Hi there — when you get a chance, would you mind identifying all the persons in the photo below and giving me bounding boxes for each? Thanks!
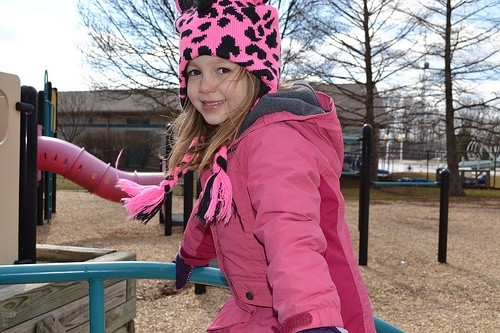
[117,1,379,333]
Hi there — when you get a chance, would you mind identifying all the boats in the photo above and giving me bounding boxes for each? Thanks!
[340,160,494,187]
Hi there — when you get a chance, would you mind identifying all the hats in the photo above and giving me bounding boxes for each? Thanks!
[115,2,281,226]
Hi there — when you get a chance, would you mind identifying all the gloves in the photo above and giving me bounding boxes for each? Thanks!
[172,254,210,295]
